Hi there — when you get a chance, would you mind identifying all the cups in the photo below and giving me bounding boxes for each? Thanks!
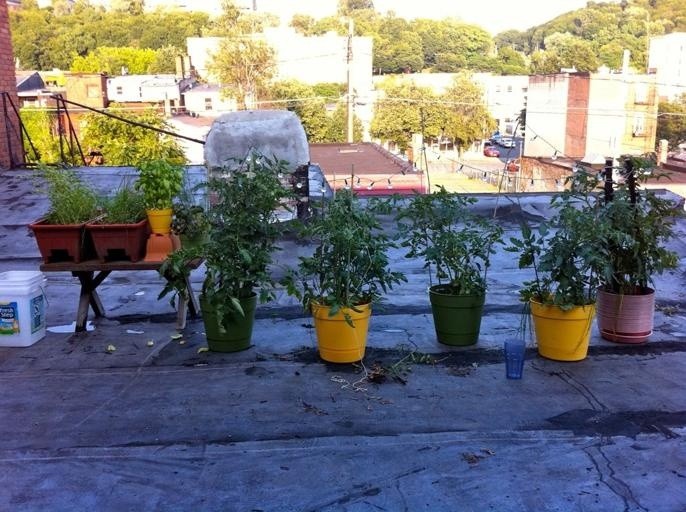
[502,336,527,380]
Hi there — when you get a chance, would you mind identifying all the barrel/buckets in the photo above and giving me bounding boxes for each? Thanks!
[429,285,484,346]
[596,286,655,344]
[530,296,596,361]
[0,271,49,348]
[310,299,372,364]
[199,291,258,352]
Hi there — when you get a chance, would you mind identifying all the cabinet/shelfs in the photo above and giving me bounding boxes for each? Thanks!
[170,201,212,249]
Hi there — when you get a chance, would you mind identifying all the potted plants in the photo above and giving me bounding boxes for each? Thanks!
[503,156,686,361]
[136,141,189,233]
[388,183,507,346]
[281,186,409,364]
[155,147,293,353]
[27,160,148,263]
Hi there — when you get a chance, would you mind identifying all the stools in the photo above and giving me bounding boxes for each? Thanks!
[39,256,203,329]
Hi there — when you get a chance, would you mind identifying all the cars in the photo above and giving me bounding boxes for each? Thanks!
[484,134,515,157]
